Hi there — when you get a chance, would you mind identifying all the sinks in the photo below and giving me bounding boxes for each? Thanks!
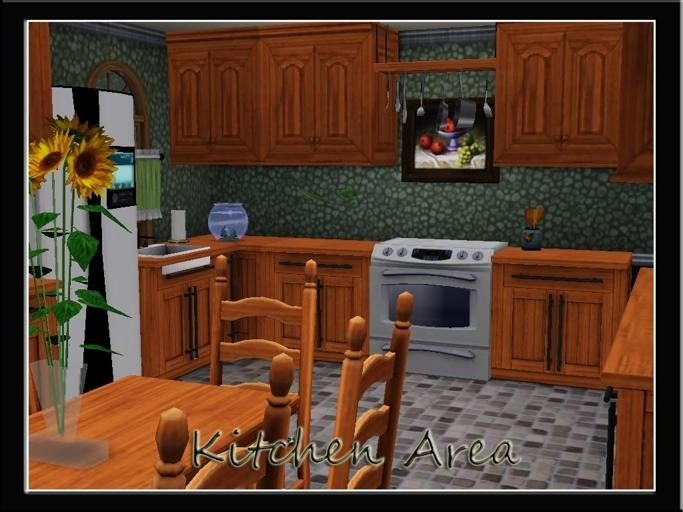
[137,243,210,258]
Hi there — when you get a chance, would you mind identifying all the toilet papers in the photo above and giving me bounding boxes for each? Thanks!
[171,210,187,241]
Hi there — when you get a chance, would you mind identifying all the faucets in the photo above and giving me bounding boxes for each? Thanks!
[137,223,140,249]
